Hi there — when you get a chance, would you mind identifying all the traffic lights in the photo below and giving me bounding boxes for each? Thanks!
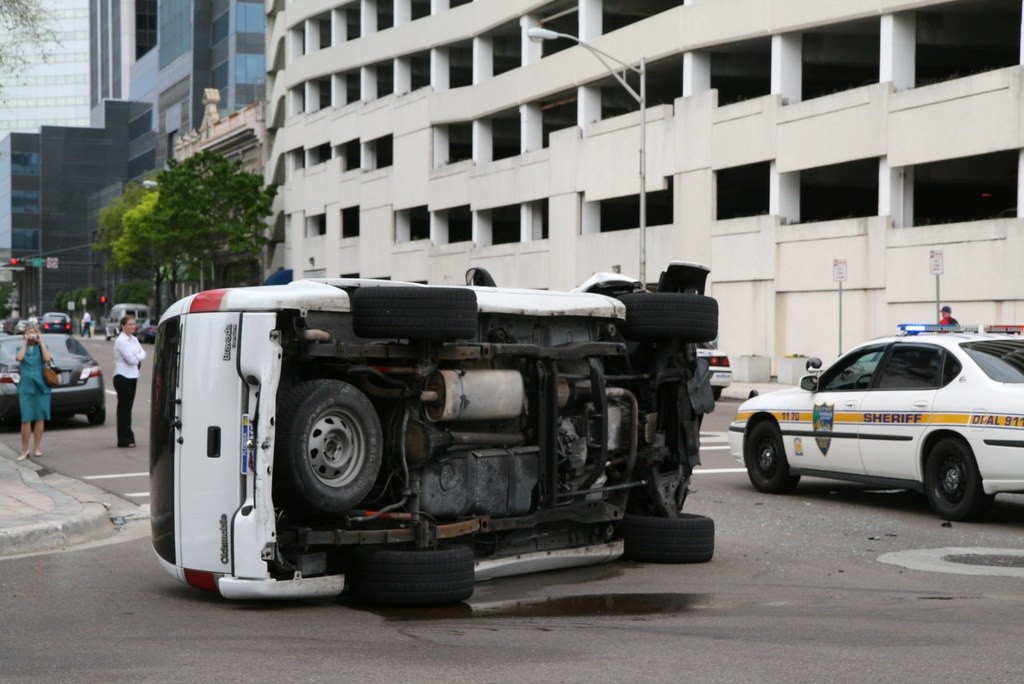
[10,258,26,266]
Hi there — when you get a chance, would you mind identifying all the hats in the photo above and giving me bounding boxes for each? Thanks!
[940,306,951,312]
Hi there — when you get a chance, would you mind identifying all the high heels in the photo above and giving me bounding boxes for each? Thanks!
[33,452,42,456]
[17,450,29,461]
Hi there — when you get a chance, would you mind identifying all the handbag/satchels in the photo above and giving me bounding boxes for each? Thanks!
[42,367,59,387]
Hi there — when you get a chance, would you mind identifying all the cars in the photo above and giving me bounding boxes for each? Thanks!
[0,334,106,432]
[729,323,1024,521]
[0,311,72,335]
[136,325,158,342]
[147,260,733,609]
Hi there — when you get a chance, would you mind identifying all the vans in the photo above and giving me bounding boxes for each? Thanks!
[105,303,151,339]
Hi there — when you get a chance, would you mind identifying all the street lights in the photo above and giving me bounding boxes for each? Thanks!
[527,28,646,295]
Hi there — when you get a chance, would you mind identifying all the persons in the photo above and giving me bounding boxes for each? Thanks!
[28,312,38,325]
[81,310,91,338]
[17,323,52,461]
[112,315,146,448]
[935,306,963,334]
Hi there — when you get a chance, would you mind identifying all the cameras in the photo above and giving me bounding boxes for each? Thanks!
[30,334,37,340]
